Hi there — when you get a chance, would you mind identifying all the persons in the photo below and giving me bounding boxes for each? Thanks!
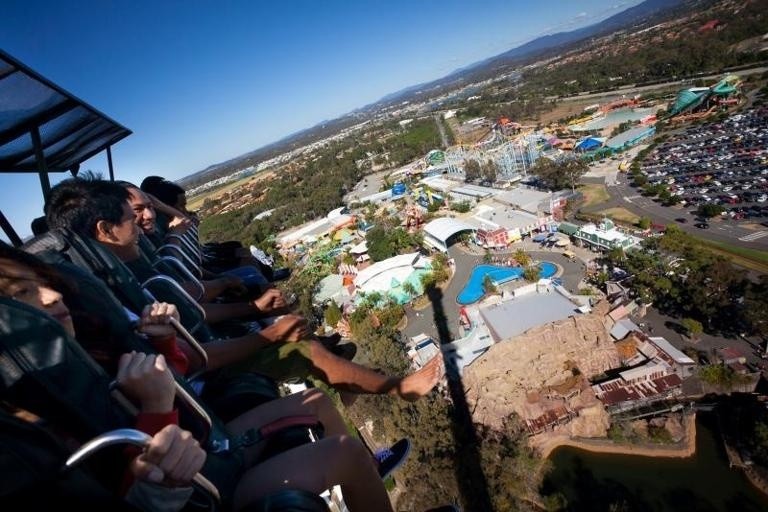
[1,173,442,511]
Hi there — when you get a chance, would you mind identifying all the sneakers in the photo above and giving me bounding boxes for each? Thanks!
[371,433,415,482]
[316,331,343,349]
[422,502,459,512]
[269,267,294,282]
[318,342,359,363]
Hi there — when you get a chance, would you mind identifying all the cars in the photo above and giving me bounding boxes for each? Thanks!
[533,231,571,250]
[585,151,631,167]
[611,268,627,282]
[638,83,768,230]
